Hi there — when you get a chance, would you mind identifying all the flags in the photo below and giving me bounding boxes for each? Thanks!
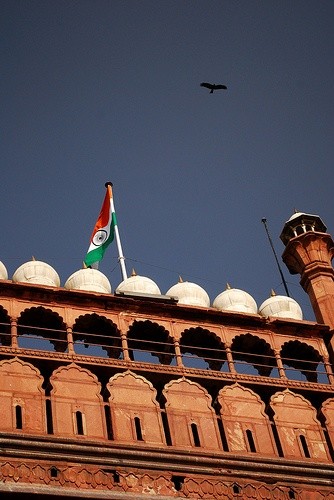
[84,184,118,270]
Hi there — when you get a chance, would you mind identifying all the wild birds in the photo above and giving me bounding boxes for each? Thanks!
[200,82,227,94]
